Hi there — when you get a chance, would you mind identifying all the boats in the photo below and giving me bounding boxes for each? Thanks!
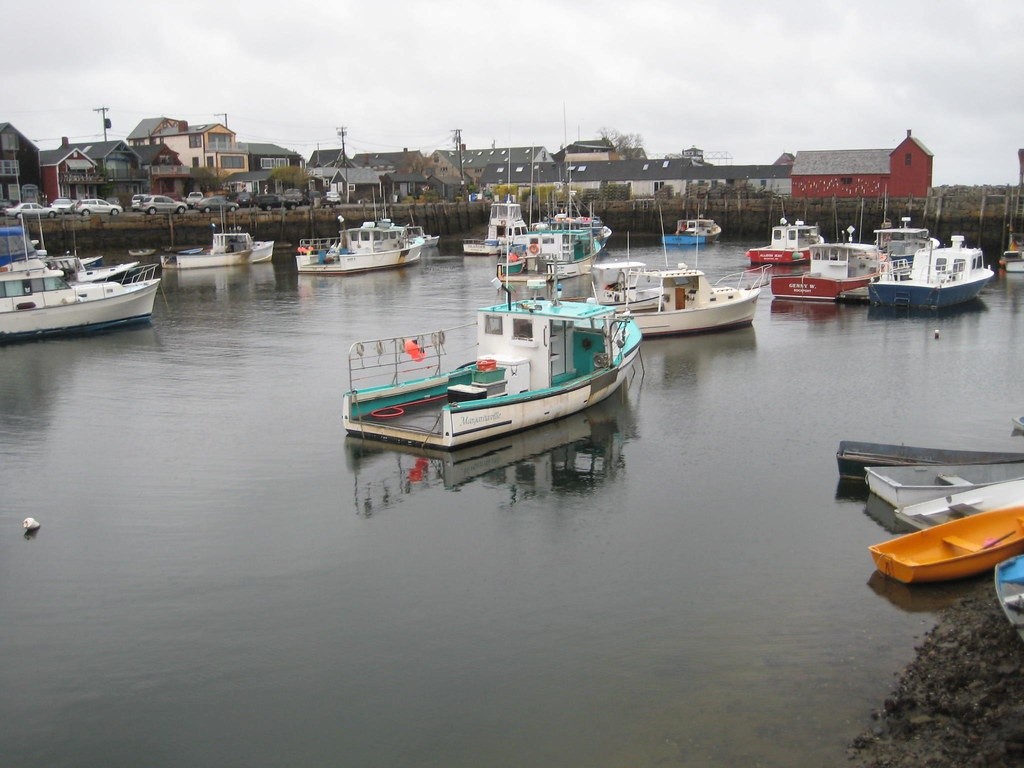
[0,257,141,288]
[836,440,1024,643]
[346,378,631,487]
[868,182,941,265]
[341,101,642,452]
[867,234,995,309]
[769,192,889,301]
[461,97,613,284]
[528,202,772,338]
[160,203,274,269]
[0,133,161,344]
[662,219,721,245]
[401,204,440,248]
[749,196,824,266]
[171,247,204,258]
[0,148,103,273]
[129,248,156,256]
[296,183,424,274]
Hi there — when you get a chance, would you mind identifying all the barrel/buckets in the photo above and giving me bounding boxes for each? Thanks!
[475,360,497,372]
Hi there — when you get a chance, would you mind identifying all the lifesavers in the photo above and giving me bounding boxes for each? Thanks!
[529,244,539,254]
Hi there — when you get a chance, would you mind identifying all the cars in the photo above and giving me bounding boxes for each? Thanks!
[0,190,342,218]
